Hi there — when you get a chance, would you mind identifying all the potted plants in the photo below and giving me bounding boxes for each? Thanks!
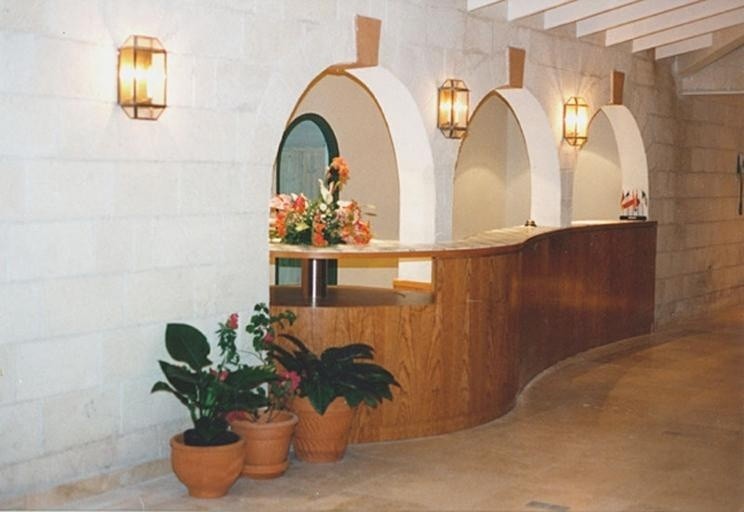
[139,317,248,497]
[272,332,403,466]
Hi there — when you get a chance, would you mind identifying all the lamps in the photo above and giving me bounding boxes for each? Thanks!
[562,95,587,145]
[114,33,170,122]
[436,78,471,141]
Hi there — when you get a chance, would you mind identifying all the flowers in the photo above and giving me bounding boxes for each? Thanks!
[264,154,373,248]
[210,297,304,425]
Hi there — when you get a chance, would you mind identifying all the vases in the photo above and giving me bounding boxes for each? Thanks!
[227,410,301,479]
